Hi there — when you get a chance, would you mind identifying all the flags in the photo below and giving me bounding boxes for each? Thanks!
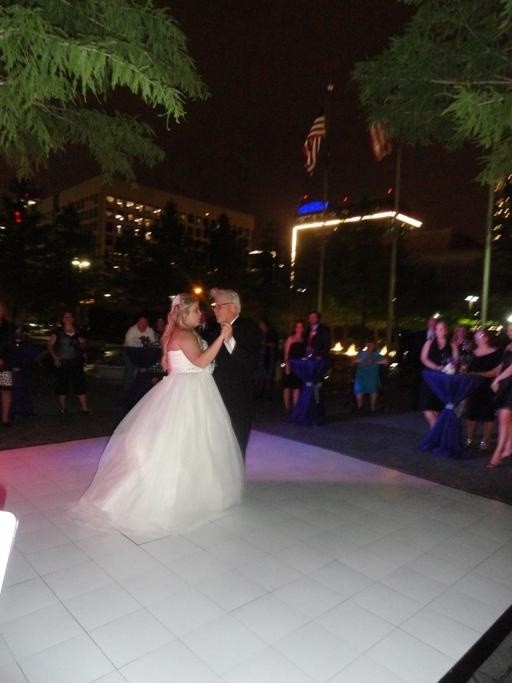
[368,117,393,160]
[303,85,326,177]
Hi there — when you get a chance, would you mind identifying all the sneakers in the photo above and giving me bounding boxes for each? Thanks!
[1,407,96,431]
[465,438,511,469]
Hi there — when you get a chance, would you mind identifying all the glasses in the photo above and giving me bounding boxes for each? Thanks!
[209,300,238,310]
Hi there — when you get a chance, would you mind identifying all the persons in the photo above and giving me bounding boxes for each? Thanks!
[1,300,20,424]
[68,291,246,535]
[417,315,511,470]
[47,310,91,415]
[122,312,389,425]
[165,289,262,468]
